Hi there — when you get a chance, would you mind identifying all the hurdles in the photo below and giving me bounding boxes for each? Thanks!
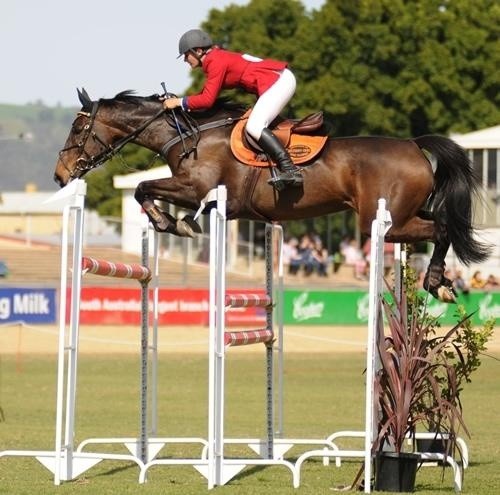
[1,178,468,493]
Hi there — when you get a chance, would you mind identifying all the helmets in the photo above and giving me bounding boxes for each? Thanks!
[177,29,212,59]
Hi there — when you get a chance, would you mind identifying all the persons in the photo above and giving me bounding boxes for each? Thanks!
[162,29,304,187]
[281,233,499,294]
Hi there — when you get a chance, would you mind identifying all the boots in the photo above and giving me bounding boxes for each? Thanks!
[256,128,304,187]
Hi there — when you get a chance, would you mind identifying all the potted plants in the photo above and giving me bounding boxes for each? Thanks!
[327,243,500,493]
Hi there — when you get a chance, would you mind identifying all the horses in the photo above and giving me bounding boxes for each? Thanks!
[53,87,497,304]
